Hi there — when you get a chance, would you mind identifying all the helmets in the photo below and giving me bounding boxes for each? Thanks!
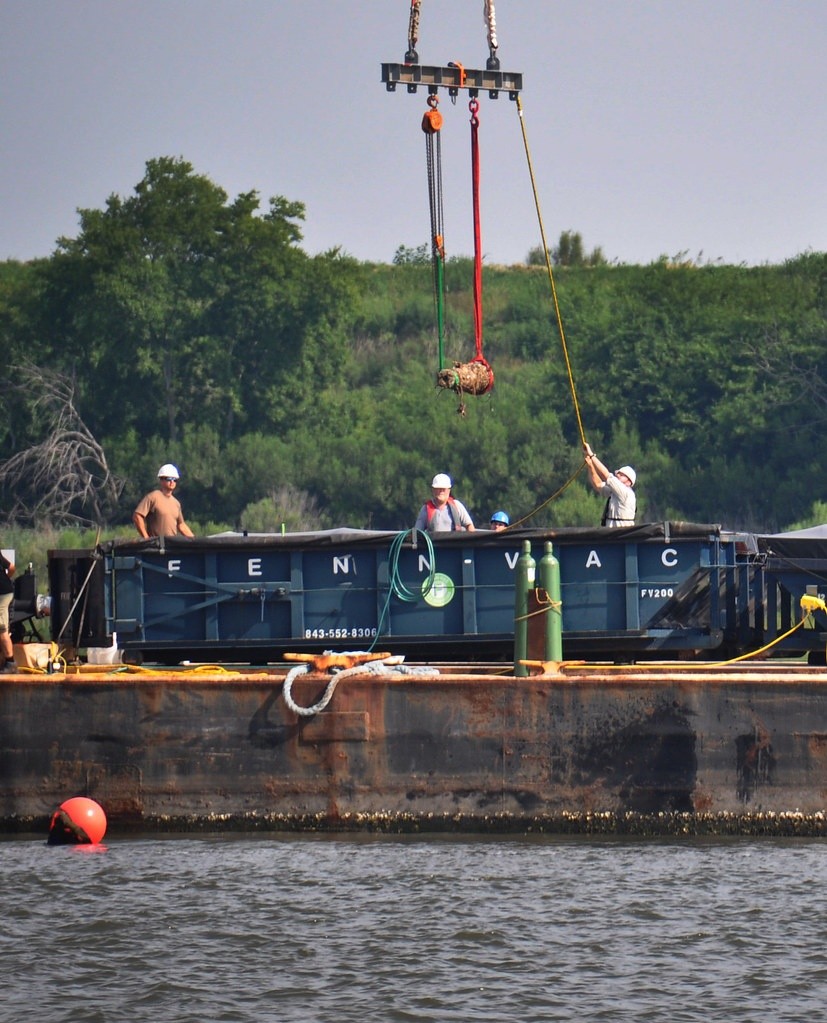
[615,465,637,487]
[490,511,509,525]
[432,473,452,489]
[157,464,180,479]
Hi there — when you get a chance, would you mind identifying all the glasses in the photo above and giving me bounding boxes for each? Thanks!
[162,477,177,482]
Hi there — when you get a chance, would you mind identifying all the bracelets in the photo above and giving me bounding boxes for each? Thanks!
[590,454,596,459]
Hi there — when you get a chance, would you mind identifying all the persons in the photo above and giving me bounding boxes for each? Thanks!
[0,551,19,674]
[491,512,509,530]
[582,443,636,527]
[414,474,475,531]
[133,464,195,537]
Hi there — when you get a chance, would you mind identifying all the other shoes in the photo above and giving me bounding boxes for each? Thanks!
[0,661,18,673]
